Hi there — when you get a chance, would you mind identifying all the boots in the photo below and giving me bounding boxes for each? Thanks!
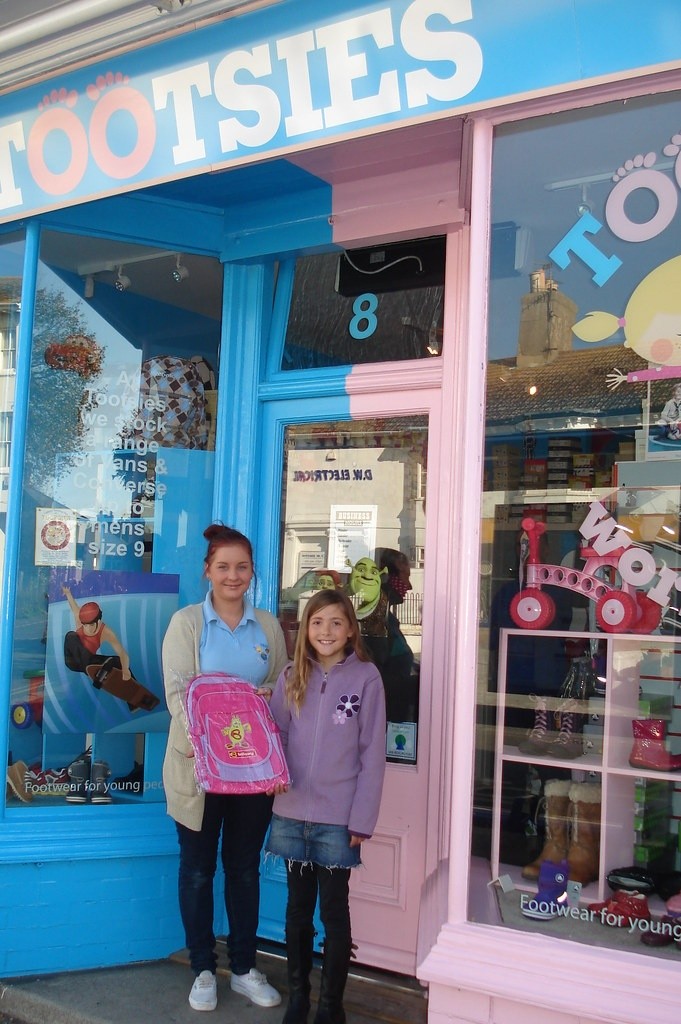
[282,930,318,1024]
[313,937,357,1024]
[499,657,681,951]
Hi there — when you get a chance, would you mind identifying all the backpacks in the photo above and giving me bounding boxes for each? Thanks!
[186,672,290,793]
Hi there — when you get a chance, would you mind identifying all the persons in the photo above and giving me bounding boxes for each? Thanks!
[358,547,417,725]
[162,521,286,1011]
[660,384,681,440]
[266,589,387,1024]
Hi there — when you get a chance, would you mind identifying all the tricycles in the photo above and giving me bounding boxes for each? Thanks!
[510,517,662,635]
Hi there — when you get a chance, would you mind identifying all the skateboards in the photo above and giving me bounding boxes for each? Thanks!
[85,656,161,711]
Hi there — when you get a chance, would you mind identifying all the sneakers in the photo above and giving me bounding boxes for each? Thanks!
[188,970,217,1010]
[231,968,281,1007]
[7,759,144,803]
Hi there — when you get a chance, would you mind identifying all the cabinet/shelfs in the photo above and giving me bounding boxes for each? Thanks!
[491,628,681,918]
[42,446,216,803]
